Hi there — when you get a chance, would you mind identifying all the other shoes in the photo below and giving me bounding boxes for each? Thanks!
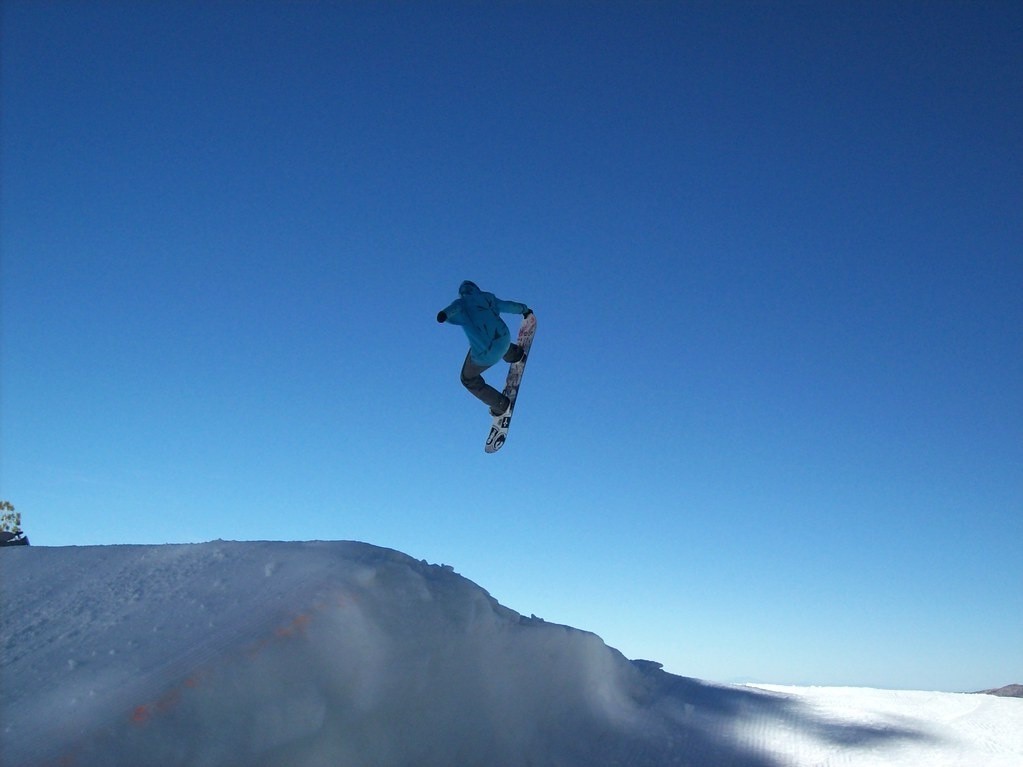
[488,407,503,417]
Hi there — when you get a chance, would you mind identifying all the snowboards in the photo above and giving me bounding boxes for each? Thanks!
[484,312,537,454]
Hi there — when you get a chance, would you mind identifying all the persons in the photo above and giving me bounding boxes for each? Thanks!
[436,279,533,420]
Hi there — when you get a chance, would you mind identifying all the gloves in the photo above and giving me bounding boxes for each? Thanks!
[437,311,448,323]
[523,308,534,319]
